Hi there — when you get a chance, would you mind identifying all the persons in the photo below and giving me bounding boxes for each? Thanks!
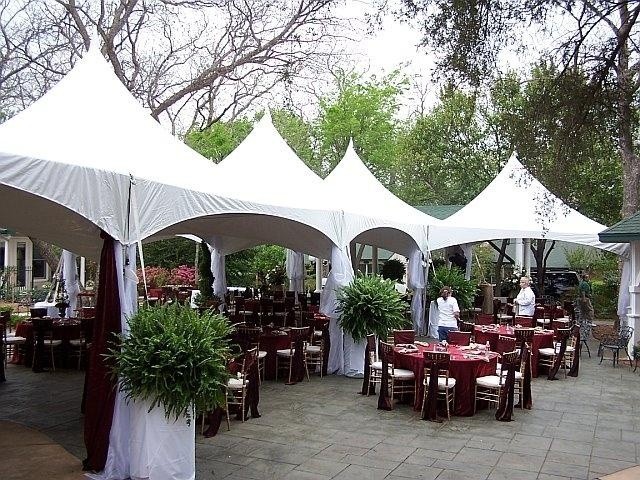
[512,276,536,317]
[575,273,598,328]
[436,285,461,341]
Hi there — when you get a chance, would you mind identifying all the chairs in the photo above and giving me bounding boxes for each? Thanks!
[0,285,196,372]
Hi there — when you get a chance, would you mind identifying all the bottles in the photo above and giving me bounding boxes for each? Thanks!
[485,340,491,352]
